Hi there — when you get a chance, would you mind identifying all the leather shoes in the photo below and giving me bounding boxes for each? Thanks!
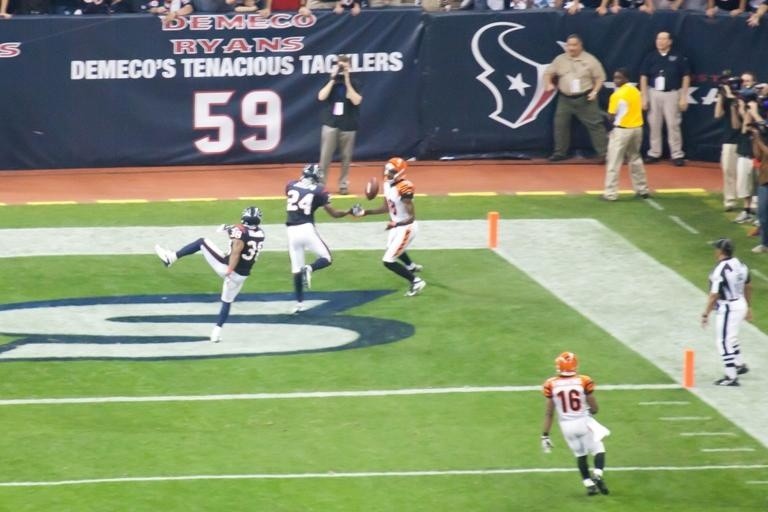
[676,159,684,166]
[595,155,606,163]
[643,155,660,163]
[548,153,569,161]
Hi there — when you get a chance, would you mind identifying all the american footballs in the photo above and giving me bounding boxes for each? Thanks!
[365,177,379,200]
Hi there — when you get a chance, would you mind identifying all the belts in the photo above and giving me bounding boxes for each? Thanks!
[654,87,678,93]
[558,88,594,100]
[611,125,625,129]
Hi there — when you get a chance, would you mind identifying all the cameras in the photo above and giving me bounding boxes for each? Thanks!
[338,65,345,73]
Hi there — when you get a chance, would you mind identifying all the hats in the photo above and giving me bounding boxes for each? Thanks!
[711,237,733,258]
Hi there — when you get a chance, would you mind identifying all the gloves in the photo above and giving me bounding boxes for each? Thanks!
[226,272,234,287]
[349,202,367,217]
[383,220,396,233]
[541,436,555,454]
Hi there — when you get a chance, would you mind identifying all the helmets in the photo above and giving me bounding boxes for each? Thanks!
[304,164,321,183]
[382,156,408,185]
[555,351,578,377]
[240,207,262,226]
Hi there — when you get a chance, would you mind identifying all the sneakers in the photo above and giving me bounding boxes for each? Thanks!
[753,219,760,227]
[732,210,753,224]
[405,275,426,297]
[748,225,762,236]
[592,473,610,495]
[715,376,740,387]
[751,244,768,254]
[292,303,306,314]
[724,362,748,376]
[583,478,600,496]
[404,263,423,274]
[153,243,176,268]
[211,326,222,343]
[300,265,312,289]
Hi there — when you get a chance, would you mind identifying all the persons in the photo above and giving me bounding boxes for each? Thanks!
[602,67,650,200]
[700,239,752,385]
[637,30,692,165]
[733,83,768,223]
[156,207,265,343]
[4,1,767,28]
[355,157,425,297]
[285,164,361,316]
[316,54,364,195]
[541,352,612,496]
[712,73,759,211]
[749,125,768,252]
[542,32,609,164]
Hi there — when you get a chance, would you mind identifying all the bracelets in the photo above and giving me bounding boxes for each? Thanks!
[329,75,337,80]
[701,314,708,318]
[543,432,549,436]
[396,222,400,226]
[590,90,600,97]
